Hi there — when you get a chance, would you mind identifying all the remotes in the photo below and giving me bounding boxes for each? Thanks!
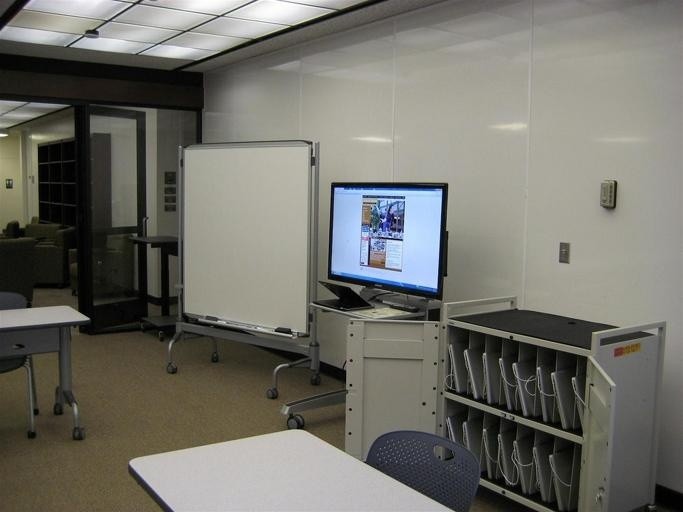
[390,302,419,313]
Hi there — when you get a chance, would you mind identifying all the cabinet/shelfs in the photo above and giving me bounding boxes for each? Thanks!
[344,293,669,512]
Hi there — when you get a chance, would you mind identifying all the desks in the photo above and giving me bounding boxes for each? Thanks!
[1,304,95,442]
[129,428,455,512]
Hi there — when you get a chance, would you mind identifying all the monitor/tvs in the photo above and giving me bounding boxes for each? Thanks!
[328,181,448,301]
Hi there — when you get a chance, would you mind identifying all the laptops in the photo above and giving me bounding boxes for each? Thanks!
[313,280,375,311]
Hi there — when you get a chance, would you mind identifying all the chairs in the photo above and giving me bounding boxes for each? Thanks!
[361,430,482,512]
[0,292,40,439]
[0,222,135,298]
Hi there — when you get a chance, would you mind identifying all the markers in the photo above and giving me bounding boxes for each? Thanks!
[205,316,218,322]
[275,326,294,335]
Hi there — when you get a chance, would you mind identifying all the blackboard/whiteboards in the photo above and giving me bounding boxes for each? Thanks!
[179,140,312,338]
